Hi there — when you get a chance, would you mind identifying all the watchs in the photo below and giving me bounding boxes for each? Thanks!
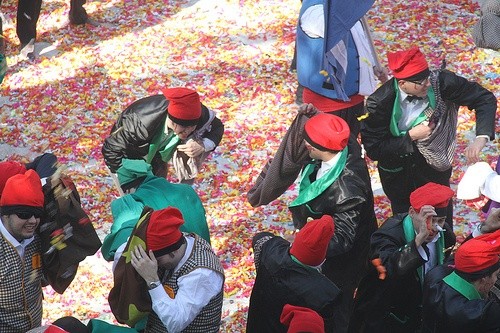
[150,281,161,290]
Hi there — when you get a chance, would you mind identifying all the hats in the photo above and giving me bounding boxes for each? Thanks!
[386,46,430,81]
[303,113,349,151]
[290,215,335,266]
[0,160,28,195]
[456,162,496,211]
[163,87,201,126]
[280,305,324,333]
[455,228,500,279]
[146,206,185,258]
[410,182,454,217]
[0,169,45,208]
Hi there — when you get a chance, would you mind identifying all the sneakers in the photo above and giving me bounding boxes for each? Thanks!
[20,42,34,59]
[84,18,101,28]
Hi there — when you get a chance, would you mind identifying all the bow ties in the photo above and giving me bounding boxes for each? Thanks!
[407,94,423,104]
[422,242,430,260]
[308,161,321,183]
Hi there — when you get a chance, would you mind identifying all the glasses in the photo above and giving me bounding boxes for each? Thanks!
[406,76,428,90]
[7,207,42,219]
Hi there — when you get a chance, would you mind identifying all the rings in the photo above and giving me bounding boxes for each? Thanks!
[138,257,141,260]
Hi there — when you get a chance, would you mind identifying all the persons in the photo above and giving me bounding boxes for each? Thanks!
[0,160,103,333]
[130,206,225,333]
[370,182,456,333]
[246,186,500,333]
[360,47,497,229]
[16,0,98,61]
[101,89,224,195]
[288,103,378,254]
[297,0,386,137]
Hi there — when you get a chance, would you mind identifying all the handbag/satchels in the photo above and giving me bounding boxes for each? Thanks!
[172,110,216,186]
[414,70,458,172]
